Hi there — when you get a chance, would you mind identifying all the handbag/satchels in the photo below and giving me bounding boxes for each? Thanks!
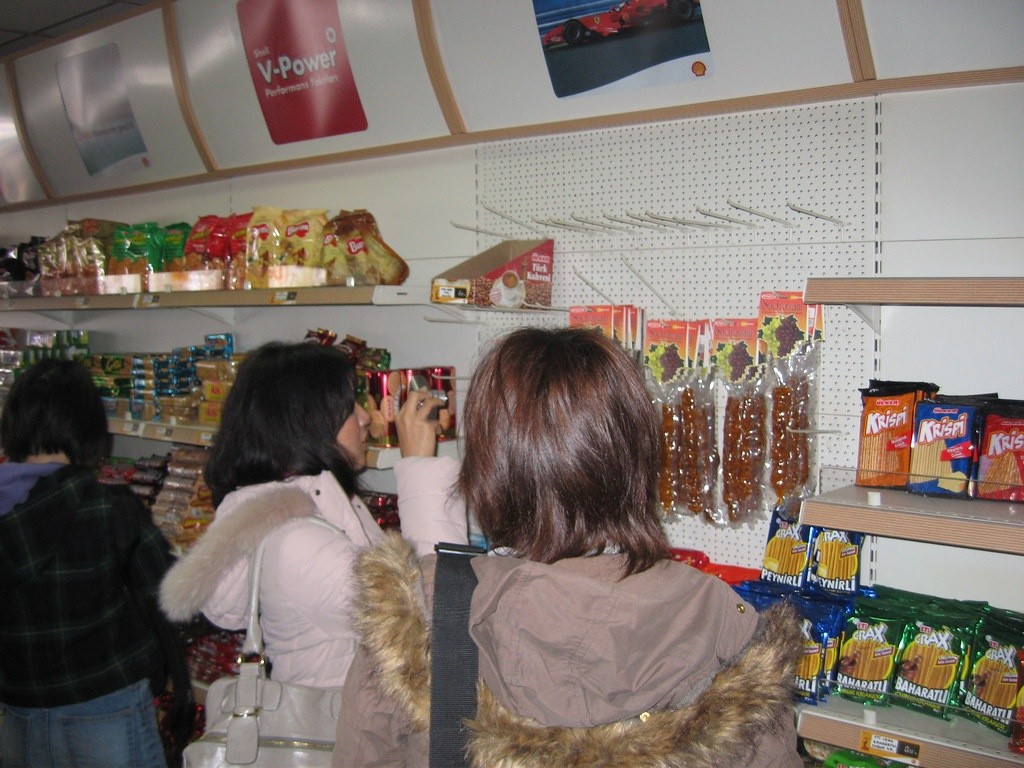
[182,515,362,768]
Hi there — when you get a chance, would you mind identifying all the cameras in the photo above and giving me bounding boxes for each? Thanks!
[408,374,449,423]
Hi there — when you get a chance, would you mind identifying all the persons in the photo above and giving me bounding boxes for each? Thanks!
[157,336,471,688]
[329,323,808,767]
[1,356,184,768]
